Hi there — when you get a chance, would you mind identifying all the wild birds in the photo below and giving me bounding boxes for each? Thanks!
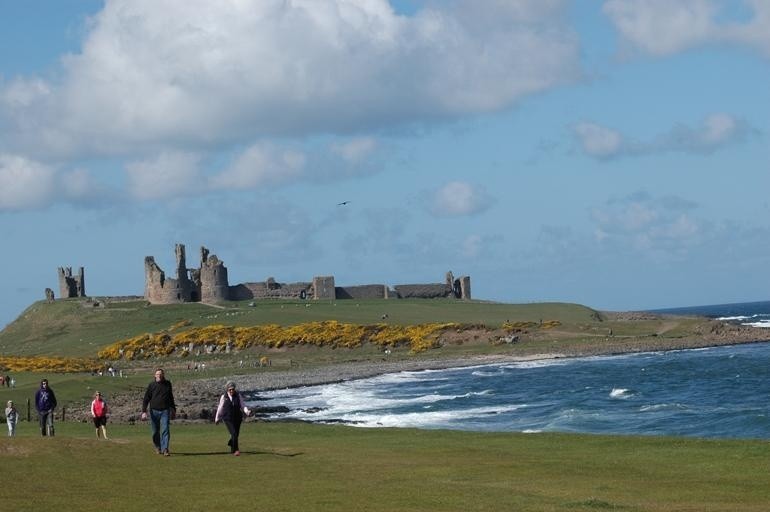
[338,201,351,205]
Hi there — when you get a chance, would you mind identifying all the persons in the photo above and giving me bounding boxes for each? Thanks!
[239,359,244,369]
[140,368,177,456]
[90,363,128,378]
[185,360,208,374]
[0,375,17,388]
[214,381,251,456]
[35,378,57,437]
[4,399,19,438]
[91,390,110,440]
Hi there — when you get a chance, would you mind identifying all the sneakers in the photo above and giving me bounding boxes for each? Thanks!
[156,448,161,454]
[164,451,170,456]
[233,451,240,456]
